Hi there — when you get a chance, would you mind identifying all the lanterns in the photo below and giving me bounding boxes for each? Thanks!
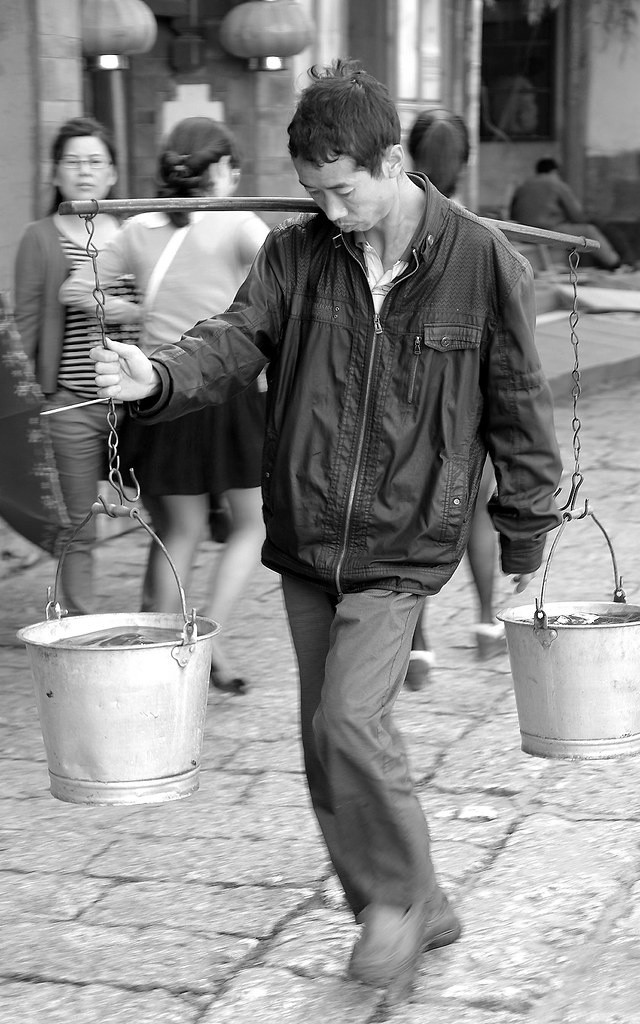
[81,0,157,71]
[219,0,313,72]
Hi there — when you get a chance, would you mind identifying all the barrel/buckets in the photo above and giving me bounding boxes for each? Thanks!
[494,504,640,761]
[15,503,222,806]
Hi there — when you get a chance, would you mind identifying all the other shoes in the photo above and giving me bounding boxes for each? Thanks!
[472,621,509,660]
[422,895,463,952]
[347,886,450,983]
[211,663,248,696]
[406,650,434,689]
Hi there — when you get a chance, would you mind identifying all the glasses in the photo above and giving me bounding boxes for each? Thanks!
[211,159,242,187]
[59,152,113,172]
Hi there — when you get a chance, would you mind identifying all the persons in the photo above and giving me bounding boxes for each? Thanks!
[508,160,627,274]
[90,58,565,992]
[57,116,271,695]
[14,118,145,619]
[406,108,508,691]
